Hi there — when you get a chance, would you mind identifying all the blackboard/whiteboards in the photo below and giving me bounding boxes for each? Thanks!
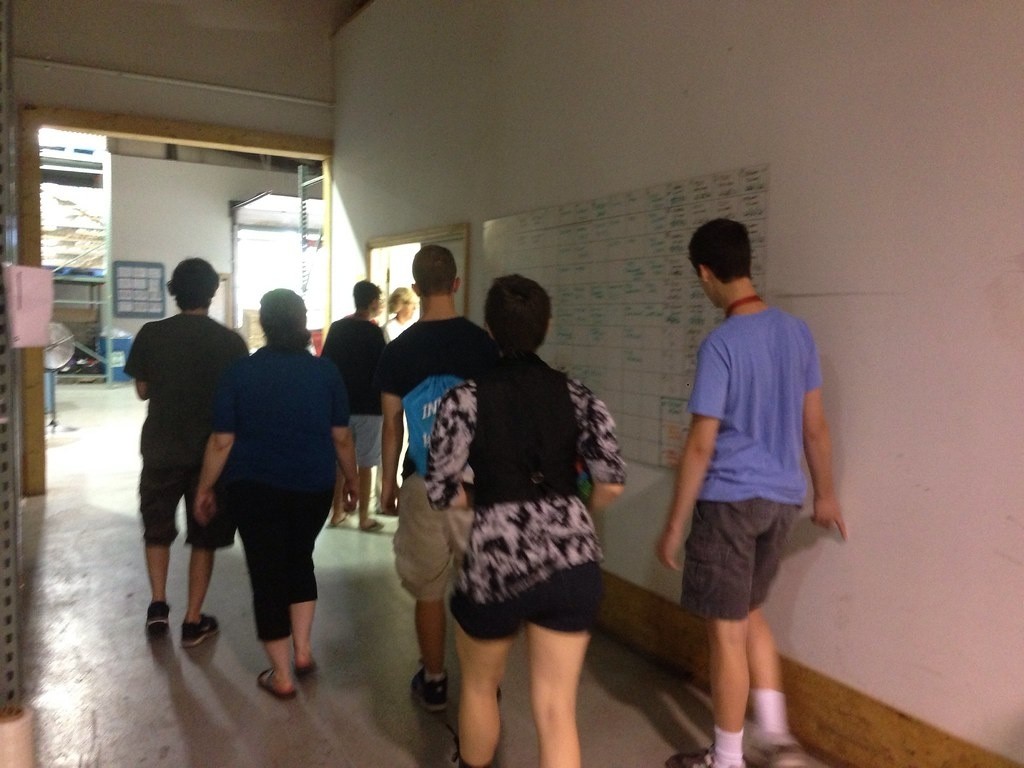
[482,163,768,474]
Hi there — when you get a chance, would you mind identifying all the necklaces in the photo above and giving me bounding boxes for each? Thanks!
[724,295,763,318]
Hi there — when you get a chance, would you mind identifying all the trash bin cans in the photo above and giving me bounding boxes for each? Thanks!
[99,337,133,382]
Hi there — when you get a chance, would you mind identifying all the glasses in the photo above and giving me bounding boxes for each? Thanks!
[375,298,384,304]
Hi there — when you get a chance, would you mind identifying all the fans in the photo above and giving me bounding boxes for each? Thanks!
[44,322,77,434]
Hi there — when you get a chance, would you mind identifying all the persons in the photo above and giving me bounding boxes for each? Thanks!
[192,288,363,699]
[377,245,505,708]
[655,218,848,768]
[425,273,626,768]
[317,279,416,533]
[121,259,249,649]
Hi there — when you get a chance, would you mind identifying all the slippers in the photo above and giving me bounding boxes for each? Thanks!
[361,519,385,531]
[327,513,352,527]
[294,657,316,677]
[258,668,297,698]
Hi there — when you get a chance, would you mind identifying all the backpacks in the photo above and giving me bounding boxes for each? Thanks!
[401,373,469,477]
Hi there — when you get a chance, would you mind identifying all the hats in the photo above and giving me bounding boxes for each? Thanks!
[258,287,307,325]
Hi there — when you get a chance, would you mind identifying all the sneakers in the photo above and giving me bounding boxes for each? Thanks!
[411,666,449,710]
[742,732,807,767]
[145,602,170,636]
[182,613,219,646]
[665,742,746,768]
